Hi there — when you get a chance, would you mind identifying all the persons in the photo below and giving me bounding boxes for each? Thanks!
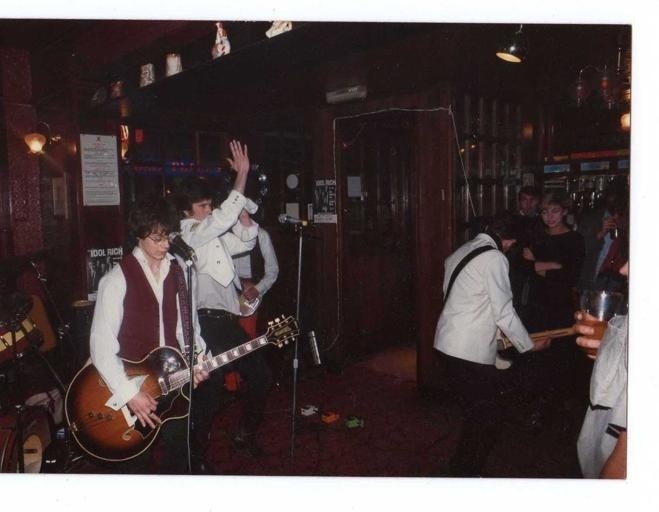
[221,176,282,396]
[170,138,276,475]
[429,176,627,479]
[87,192,211,474]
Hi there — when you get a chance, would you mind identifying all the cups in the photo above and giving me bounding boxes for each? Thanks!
[577,289,625,360]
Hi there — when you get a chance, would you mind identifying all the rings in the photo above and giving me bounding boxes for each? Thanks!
[148,411,154,416]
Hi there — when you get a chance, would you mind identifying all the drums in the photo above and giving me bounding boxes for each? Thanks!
[1,352,67,430]
[0,400,60,473]
[0,297,44,368]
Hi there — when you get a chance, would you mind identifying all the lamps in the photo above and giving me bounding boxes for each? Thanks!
[568,33,631,134]
[496,24,530,64]
[24,122,62,157]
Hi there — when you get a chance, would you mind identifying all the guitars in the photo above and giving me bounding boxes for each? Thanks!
[64,311,304,461]
[495,309,594,370]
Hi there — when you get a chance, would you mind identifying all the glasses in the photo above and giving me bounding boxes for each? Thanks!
[147,234,167,245]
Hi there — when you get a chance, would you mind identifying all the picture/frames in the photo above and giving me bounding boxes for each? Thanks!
[51,169,69,221]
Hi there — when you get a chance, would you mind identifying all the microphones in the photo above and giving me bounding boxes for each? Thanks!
[168,232,199,262]
[278,214,308,227]
